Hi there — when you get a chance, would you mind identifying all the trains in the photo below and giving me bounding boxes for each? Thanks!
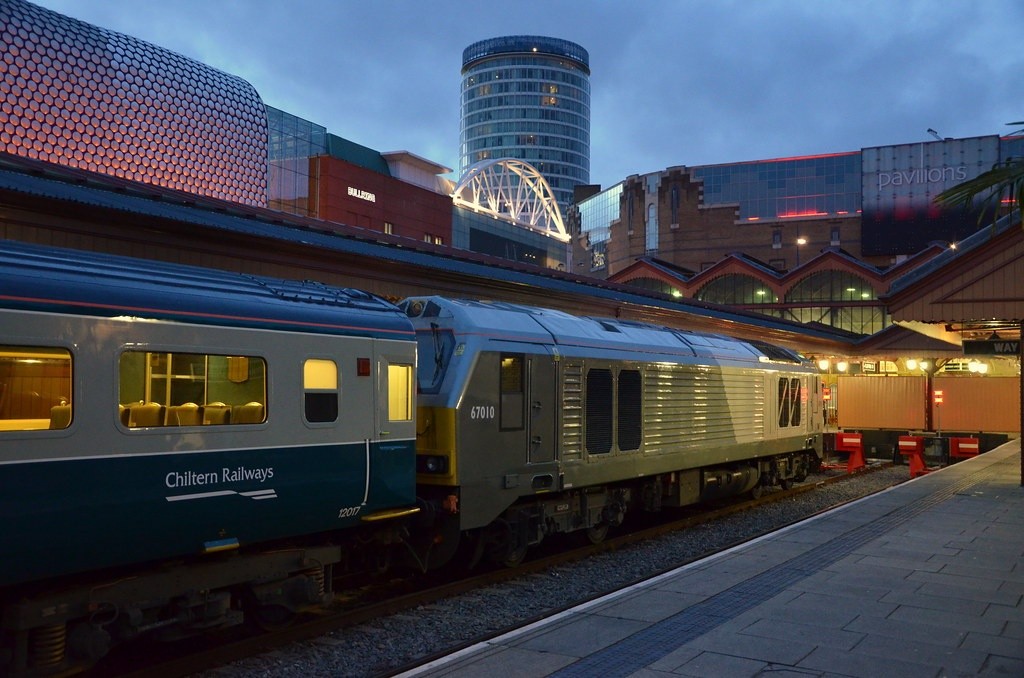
[1,241,826,678]
